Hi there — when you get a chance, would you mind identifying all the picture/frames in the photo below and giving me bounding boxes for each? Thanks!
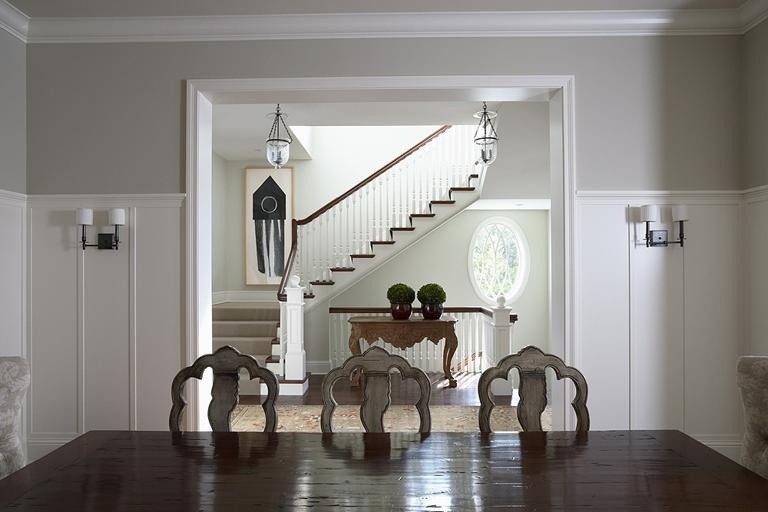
[243,166,295,285]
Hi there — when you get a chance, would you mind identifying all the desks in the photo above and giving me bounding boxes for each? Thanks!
[0,430,766,512]
[348,316,458,388]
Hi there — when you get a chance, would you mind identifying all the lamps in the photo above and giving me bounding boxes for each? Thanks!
[265,104,293,167]
[77,208,125,251]
[473,101,499,165]
[639,204,689,247]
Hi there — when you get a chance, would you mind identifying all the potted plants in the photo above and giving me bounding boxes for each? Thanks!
[387,283,414,319]
[417,283,446,319]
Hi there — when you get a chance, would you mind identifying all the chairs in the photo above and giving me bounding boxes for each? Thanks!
[477,346,590,433]
[319,346,431,433]
[0,355,32,478]
[735,356,767,479]
[168,345,278,434]
